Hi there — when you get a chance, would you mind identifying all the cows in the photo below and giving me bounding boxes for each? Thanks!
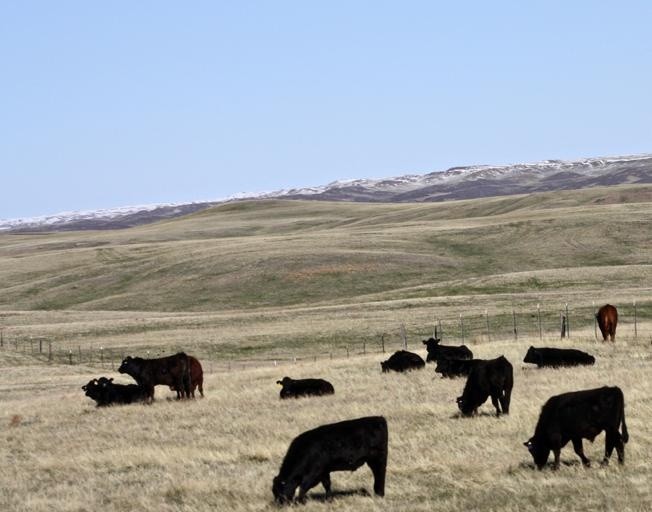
[522,385,629,471]
[270,414,389,505]
[80,352,206,409]
[380,349,426,373]
[456,355,515,417]
[275,376,335,399]
[595,303,618,341]
[435,358,489,379]
[422,337,474,363]
[522,345,596,368]
